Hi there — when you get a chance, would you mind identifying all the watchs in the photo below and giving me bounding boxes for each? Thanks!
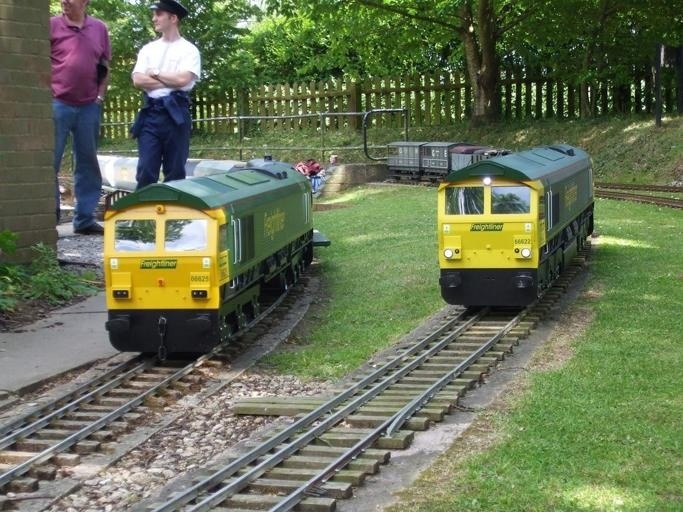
[97,95,105,102]
[153,69,160,78]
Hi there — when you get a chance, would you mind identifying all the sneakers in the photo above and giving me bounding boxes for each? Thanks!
[75,222,104,235]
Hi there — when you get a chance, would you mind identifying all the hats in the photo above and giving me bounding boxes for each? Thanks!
[147,0,189,20]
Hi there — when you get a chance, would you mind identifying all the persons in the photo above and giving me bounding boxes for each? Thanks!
[48,0,107,240]
[126,0,202,192]
